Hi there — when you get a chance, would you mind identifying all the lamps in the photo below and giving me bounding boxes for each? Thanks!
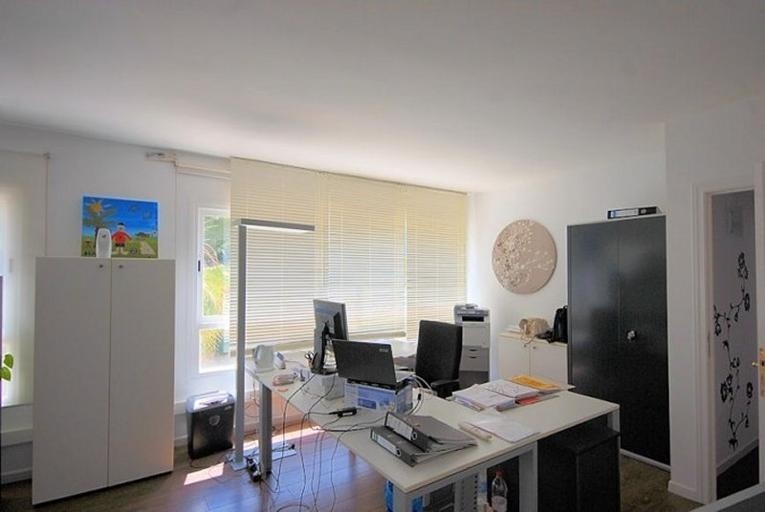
[226,214,319,473]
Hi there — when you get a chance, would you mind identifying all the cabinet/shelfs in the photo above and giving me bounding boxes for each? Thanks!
[493,329,569,382]
[29,247,181,509]
[479,417,622,511]
[454,319,492,390]
[563,211,676,475]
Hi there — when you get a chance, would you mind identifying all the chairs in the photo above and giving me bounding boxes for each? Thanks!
[410,316,466,400]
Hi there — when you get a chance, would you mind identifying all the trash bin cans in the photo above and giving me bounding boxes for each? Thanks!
[370,411,478,467]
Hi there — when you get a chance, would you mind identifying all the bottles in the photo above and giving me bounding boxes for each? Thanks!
[487,469,509,510]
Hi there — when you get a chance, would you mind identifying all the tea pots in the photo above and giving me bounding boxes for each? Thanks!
[251,345,276,371]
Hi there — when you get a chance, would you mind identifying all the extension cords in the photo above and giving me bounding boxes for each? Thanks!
[248,458,259,482]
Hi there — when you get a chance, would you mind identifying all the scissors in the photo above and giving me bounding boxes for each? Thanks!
[304,351,315,361]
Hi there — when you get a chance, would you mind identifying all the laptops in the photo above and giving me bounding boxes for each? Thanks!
[332,339,416,385]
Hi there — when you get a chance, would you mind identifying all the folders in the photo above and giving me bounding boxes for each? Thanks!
[607,206,662,218]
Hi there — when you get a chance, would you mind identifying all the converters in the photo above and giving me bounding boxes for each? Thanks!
[337,407,357,417]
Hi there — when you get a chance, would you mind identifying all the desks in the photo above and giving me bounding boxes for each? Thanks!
[246,345,628,512]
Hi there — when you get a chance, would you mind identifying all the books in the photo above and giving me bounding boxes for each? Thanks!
[452,375,576,412]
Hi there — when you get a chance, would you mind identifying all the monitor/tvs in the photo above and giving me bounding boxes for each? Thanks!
[311,299,348,375]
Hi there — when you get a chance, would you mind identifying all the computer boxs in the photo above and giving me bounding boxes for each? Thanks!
[186,389,235,460]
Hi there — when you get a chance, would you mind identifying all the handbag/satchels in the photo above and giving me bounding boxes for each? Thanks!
[553,305,568,343]
[520,318,550,335]
